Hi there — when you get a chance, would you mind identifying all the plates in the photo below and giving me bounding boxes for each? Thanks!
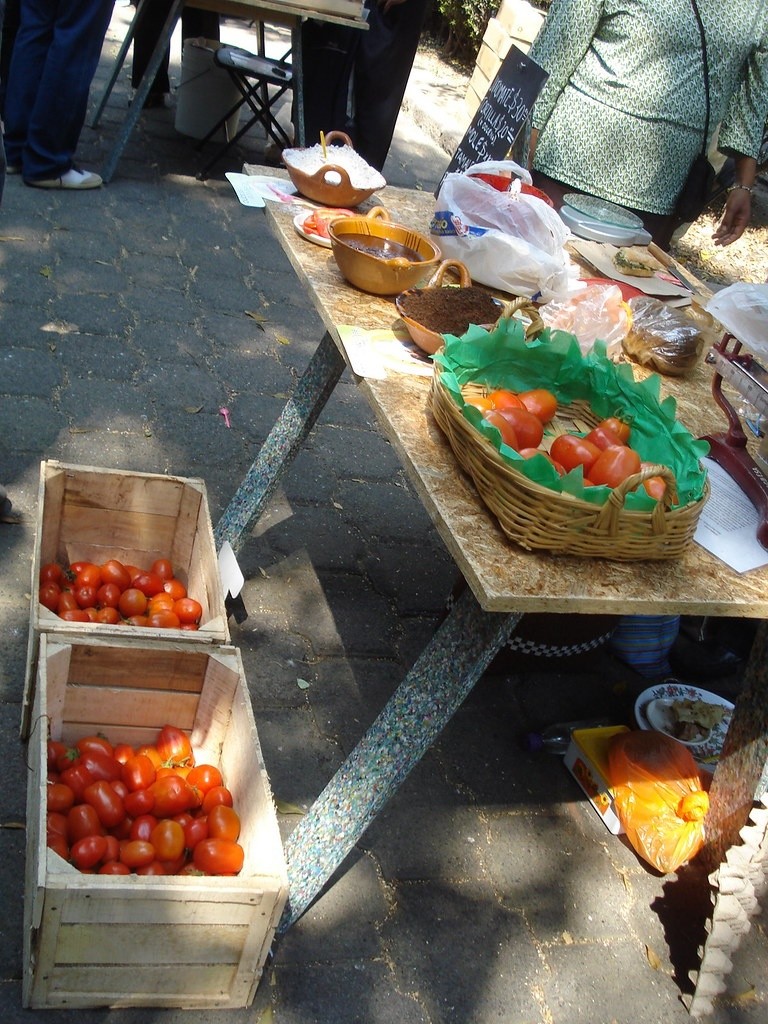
[635,684,735,763]
[648,699,712,745]
[293,213,358,248]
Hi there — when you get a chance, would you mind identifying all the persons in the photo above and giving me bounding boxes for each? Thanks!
[512,0,768,253]
[128,0,220,109]
[0,0,116,189]
[264,0,434,172]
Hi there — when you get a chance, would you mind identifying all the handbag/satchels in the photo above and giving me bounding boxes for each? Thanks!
[677,159,716,223]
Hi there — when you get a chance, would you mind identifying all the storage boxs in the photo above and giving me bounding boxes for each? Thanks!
[16,457,231,745]
[18,631,289,1008]
[566,725,634,836]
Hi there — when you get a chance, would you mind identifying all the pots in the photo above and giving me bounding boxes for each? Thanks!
[397,259,525,357]
[283,131,387,207]
[327,206,443,296]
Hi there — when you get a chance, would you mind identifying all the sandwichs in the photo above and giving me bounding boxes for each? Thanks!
[613,247,659,277]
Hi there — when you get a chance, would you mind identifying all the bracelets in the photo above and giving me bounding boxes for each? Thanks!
[727,184,753,195]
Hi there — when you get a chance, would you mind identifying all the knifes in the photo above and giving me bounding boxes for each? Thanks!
[648,244,702,295]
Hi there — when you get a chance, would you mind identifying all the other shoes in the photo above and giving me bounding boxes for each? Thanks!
[135,93,170,108]
[5,162,104,191]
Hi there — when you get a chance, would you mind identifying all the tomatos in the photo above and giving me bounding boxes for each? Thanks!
[304,206,358,240]
[462,389,682,507]
[44,724,246,877]
[39,558,203,632]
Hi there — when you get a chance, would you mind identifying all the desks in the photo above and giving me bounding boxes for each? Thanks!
[92,0,369,187]
[215,161,767,937]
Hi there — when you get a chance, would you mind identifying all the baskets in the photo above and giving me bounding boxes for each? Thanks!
[426,296,712,562]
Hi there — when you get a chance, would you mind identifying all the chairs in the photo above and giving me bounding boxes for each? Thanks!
[196,46,293,182]
